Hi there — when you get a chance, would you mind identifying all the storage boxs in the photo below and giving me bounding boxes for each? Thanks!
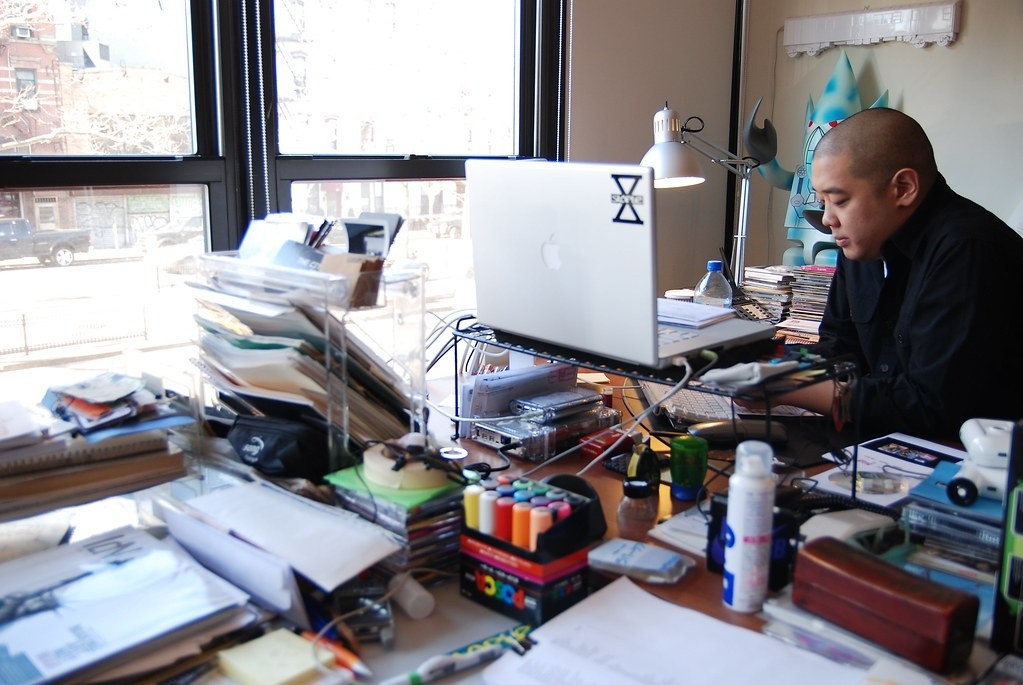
[580,424,650,462]
[459,534,590,627]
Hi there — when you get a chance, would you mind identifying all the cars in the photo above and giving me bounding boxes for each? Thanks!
[141,212,211,276]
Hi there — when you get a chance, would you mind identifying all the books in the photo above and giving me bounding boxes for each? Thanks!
[0,524,278,684]
[322,459,466,584]
[188,281,419,450]
[742,263,837,344]
[0,400,187,523]
[657,297,738,328]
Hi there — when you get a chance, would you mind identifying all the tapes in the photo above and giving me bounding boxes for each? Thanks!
[362,436,446,489]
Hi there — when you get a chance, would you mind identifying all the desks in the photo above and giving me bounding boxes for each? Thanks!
[426,357,839,634]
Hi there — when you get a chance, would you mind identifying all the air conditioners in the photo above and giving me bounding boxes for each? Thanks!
[13,26,30,38]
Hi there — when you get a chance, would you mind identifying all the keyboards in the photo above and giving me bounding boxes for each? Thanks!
[636,376,742,425]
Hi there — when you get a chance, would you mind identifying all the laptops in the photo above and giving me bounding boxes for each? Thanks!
[465,156,777,371]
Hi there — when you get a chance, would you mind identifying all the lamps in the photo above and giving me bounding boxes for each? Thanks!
[639,101,753,286]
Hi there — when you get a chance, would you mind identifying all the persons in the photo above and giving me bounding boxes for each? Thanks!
[733,107,1023,441]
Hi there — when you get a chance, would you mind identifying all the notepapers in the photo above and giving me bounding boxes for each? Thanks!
[212,629,335,685]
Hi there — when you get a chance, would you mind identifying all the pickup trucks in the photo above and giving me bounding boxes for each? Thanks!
[0,217,94,267]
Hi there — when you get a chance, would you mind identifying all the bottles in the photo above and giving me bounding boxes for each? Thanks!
[669,434,708,501]
[720,441,776,613]
[617,477,658,543]
[693,260,733,311]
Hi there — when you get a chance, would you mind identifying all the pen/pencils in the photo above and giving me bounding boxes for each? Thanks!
[275,620,377,677]
[379,646,505,685]
